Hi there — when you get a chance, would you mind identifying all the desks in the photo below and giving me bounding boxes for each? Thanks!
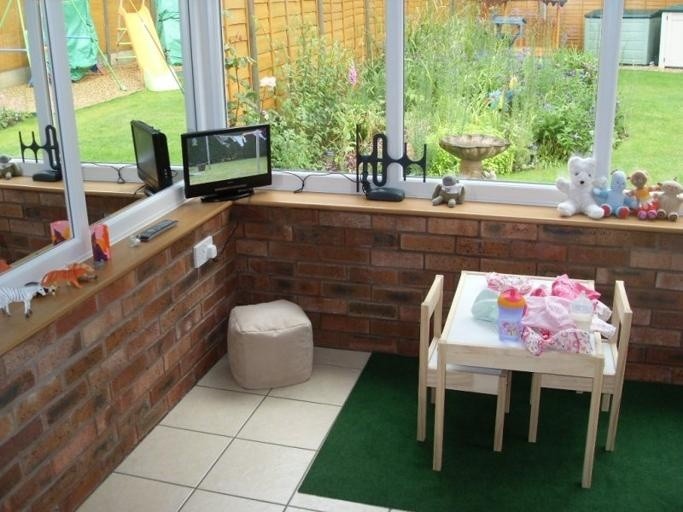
[432,268,605,491]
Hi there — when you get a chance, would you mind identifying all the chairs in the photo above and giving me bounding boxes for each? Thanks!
[417,273,514,452]
[528,279,632,454]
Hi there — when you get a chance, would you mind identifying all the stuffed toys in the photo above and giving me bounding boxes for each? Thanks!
[625,171,663,220]
[654,177,683,221]
[0,155,20,179]
[593,171,637,219]
[432,175,464,208]
[557,157,608,219]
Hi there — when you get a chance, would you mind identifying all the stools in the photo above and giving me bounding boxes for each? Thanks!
[229,298,314,390]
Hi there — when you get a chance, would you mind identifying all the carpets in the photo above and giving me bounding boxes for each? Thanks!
[296,349,682,512]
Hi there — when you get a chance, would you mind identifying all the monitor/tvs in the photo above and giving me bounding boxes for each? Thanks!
[182,125,271,202]
[130,119,173,199]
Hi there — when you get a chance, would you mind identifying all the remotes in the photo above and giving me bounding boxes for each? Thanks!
[134,216,179,243]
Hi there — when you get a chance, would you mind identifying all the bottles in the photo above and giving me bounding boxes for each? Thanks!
[496,286,525,345]
[569,289,595,333]
